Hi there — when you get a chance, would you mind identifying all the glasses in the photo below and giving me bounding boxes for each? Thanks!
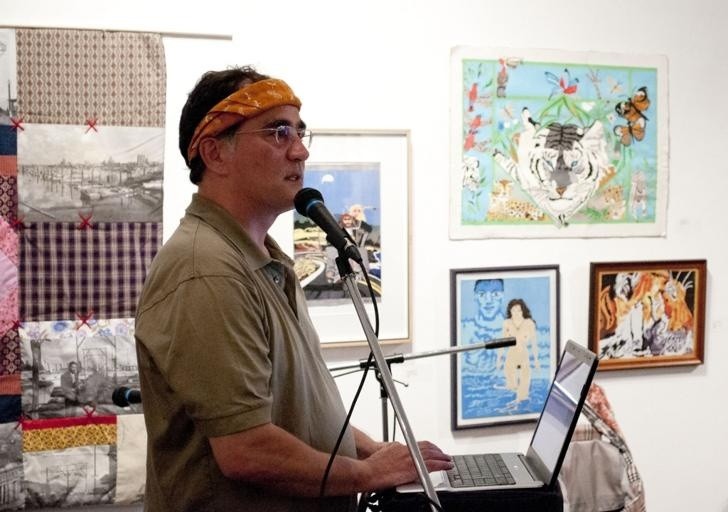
[240,126,312,149]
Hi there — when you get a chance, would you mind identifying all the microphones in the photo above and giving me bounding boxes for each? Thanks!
[293,187,363,264]
[112,386,142,408]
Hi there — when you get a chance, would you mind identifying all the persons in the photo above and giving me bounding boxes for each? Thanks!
[496,299,540,401]
[461,279,505,374]
[134,69,454,512]
[60,362,80,417]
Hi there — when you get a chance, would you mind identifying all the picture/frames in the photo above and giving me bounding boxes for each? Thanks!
[451,264,560,431]
[449,46,669,240]
[588,259,707,372]
[267,129,413,349]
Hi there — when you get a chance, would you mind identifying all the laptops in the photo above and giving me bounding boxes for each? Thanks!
[395,339,599,496]
